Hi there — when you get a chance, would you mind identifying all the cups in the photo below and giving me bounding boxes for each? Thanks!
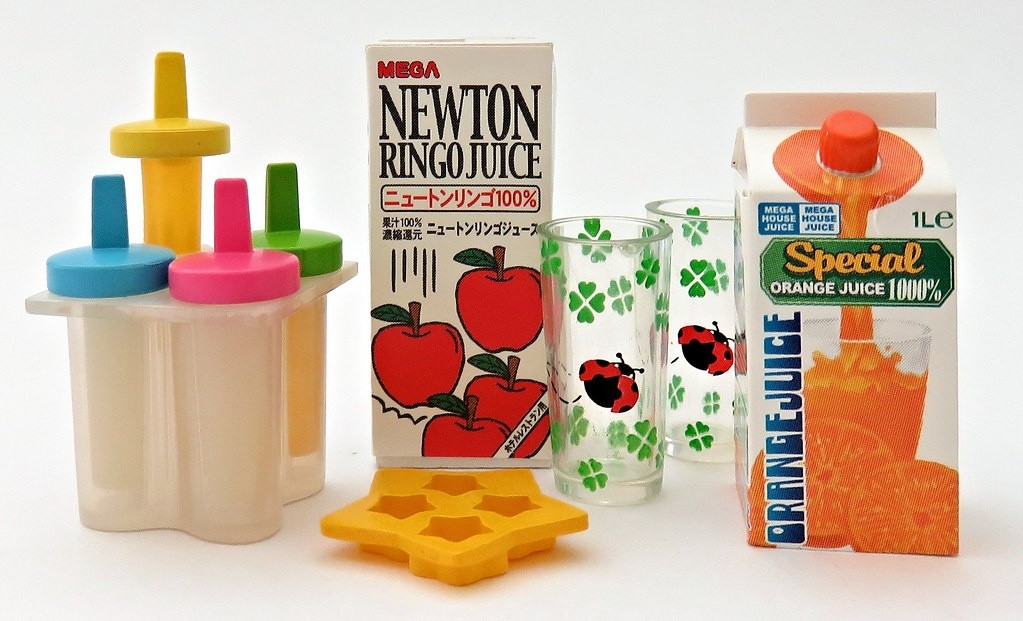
[644,197,743,464]
[535,215,673,508]
